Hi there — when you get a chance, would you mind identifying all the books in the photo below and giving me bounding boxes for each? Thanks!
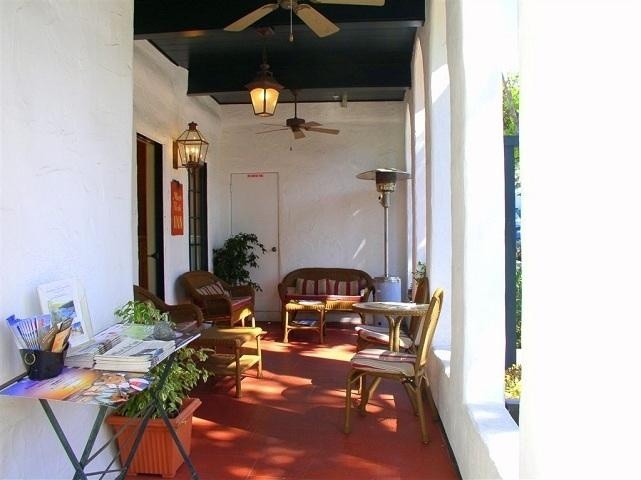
[64,324,201,373]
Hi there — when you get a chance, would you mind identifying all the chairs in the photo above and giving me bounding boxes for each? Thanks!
[340,288,445,445]
[179,270,256,327]
[353,276,430,401]
[133,284,204,333]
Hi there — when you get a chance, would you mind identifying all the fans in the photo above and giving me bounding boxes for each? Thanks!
[252,90,340,141]
[222,1,385,43]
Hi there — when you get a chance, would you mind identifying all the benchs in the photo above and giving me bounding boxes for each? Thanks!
[276,266,373,336]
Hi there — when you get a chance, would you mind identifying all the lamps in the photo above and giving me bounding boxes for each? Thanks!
[170,121,210,170]
[353,166,415,330]
[243,27,285,118]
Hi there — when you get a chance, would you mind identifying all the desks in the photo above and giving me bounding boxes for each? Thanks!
[352,300,430,418]
[0,322,203,480]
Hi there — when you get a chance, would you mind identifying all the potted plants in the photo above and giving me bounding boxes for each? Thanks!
[102,298,203,480]
[213,231,268,325]
